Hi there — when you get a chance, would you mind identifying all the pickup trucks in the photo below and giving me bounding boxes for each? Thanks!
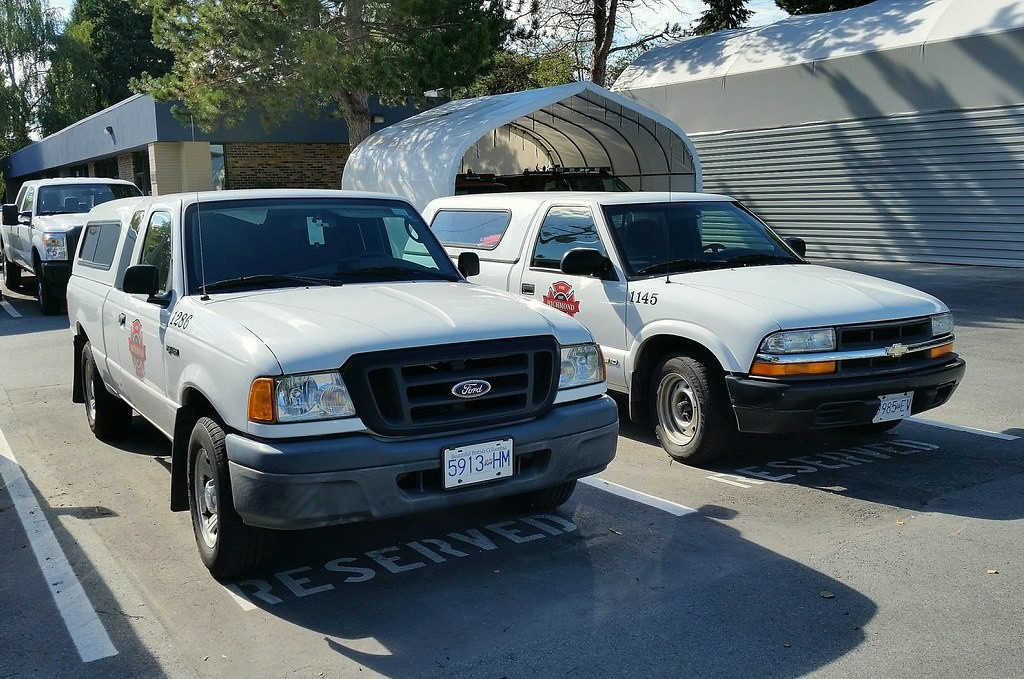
[403,191,965,469]
[65,188,619,580]
[0,178,152,318]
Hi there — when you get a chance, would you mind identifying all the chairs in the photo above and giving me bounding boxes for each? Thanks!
[43,192,65,211]
[62,197,81,211]
[621,219,664,263]
